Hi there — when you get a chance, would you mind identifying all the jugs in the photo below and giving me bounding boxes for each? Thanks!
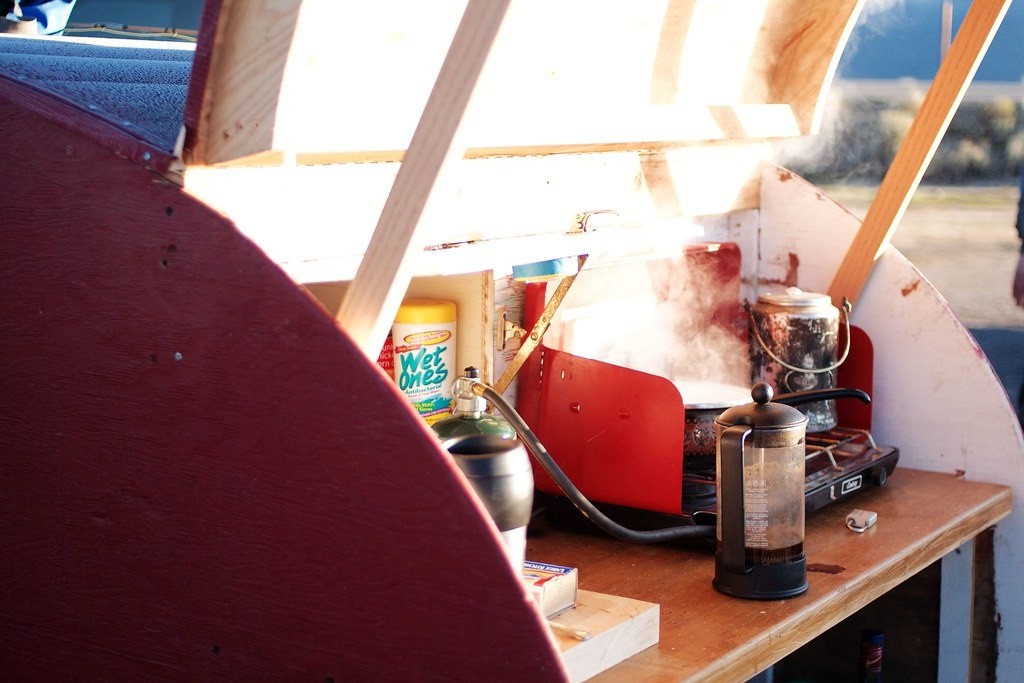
[745,287,852,433]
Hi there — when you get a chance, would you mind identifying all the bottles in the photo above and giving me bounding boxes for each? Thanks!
[858,631,884,683]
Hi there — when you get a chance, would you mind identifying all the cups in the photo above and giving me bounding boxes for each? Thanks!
[710,383,809,601]
[441,435,535,532]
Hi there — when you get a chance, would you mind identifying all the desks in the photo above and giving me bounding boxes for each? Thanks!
[524,467,1014,683]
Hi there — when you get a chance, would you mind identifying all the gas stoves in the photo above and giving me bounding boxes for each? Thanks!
[681,427,900,526]
